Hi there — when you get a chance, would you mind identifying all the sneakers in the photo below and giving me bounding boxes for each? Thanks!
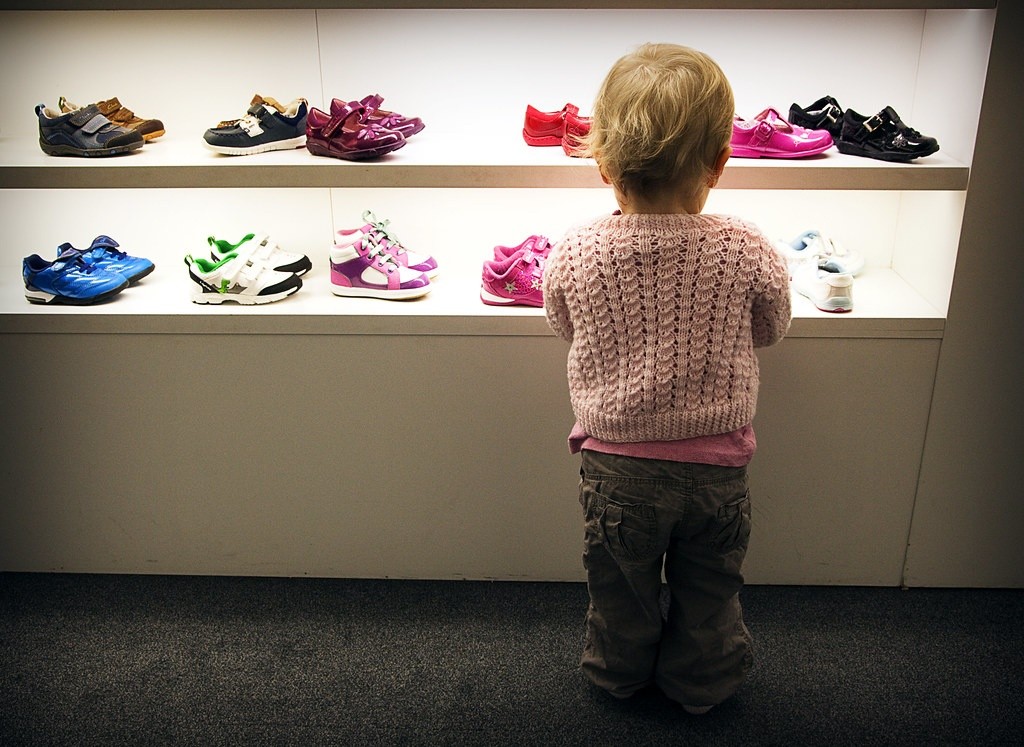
[330,210,439,300]
[184,233,311,305]
[35,96,165,158]
[783,229,872,312]
[205,97,306,155]
[22,236,154,305]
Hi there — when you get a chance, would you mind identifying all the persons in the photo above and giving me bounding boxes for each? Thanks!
[543,44,792,713]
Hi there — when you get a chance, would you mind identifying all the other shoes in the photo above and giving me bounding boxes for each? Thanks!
[604,686,716,718]
[480,236,553,307]
[523,103,594,158]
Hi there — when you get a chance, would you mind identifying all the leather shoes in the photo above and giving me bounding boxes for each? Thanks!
[728,108,833,158]
[789,96,940,159]
[306,94,424,158]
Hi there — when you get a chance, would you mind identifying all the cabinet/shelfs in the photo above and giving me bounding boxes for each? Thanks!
[0,0,1005,339]
